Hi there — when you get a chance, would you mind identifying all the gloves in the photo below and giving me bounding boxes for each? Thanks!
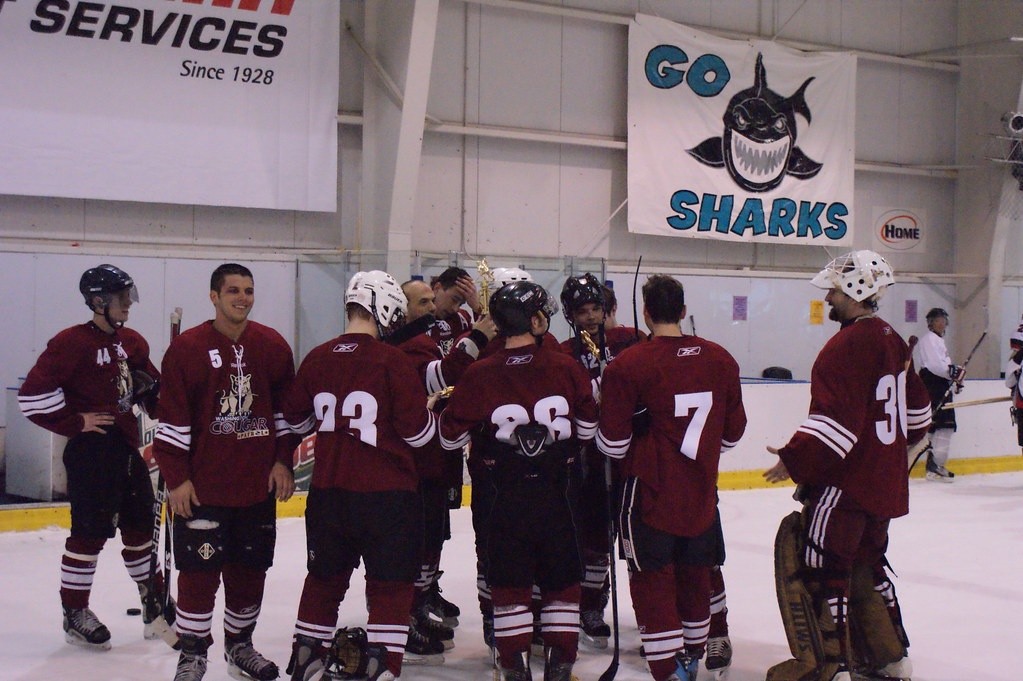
[949,365,966,383]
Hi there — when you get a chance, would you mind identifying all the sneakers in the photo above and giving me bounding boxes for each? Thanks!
[410,592,455,649]
[62,603,111,649]
[401,616,445,666]
[830,663,852,681]
[705,635,732,677]
[224,622,279,681]
[870,656,912,681]
[925,460,954,482]
[578,608,611,648]
[544,648,573,681]
[497,651,531,681]
[530,620,579,660]
[426,570,460,628]
[138,583,179,639]
[173,634,213,681]
[484,616,495,647]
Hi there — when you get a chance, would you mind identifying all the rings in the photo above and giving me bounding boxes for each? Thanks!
[781,476,785,480]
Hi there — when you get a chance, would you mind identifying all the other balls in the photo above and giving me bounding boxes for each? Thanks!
[127,608,141,616]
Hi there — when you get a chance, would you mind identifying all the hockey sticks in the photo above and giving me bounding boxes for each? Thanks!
[941,396,1013,410]
[574,323,619,681]
[146,307,182,651]
[904,335,918,374]
[385,313,437,347]
[932,330,988,418]
[601,257,606,283]
[690,315,696,336]
[633,255,642,342]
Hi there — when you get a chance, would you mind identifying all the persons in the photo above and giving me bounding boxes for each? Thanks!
[595,274,747,681]
[282,271,445,681]
[914,308,966,483]
[438,281,600,681]
[765,249,932,681]
[394,266,649,665]
[18,264,176,651]
[1005,312,1023,455]
[152,263,304,681]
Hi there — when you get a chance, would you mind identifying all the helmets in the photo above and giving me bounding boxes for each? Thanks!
[496,268,532,292]
[344,270,411,340]
[80,264,133,305]
[323,626,369,678]
[489,282,547,330]
[926,308,949,318]
[560,273,605,311]
[810,249,896,303]
